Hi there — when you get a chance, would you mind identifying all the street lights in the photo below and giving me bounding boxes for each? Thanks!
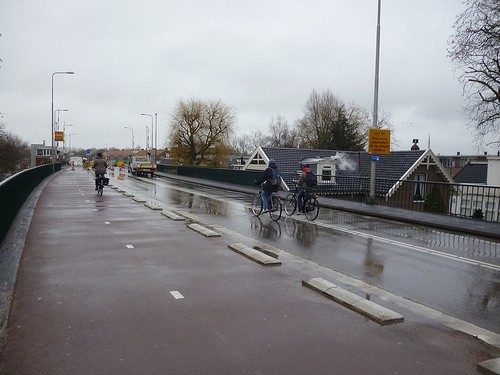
[52,78,77,174]
[140,114,153,149]
[124,126,134,157]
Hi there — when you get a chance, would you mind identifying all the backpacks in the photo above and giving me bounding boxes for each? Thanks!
[307,172,317,187]
[269,167,281,186]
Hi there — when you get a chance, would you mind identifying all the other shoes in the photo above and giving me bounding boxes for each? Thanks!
[95,188,98,190]
[262,209,269,212]
[297,212,303,215]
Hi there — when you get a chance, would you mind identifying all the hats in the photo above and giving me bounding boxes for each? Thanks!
[302,164,309,167]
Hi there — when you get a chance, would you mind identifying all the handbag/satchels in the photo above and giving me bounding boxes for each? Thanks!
[103,178,108,185]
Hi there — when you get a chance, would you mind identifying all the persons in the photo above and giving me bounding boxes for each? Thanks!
[255,159,281,214]
[92,153,108,191]
[292,163,317,213]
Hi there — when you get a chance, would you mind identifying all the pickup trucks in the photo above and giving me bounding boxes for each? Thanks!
[128,156,157,177]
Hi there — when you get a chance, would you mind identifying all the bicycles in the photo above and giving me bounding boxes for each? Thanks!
[251,180,283,221]
[284,180,319,221]
[92,167,107,196]
